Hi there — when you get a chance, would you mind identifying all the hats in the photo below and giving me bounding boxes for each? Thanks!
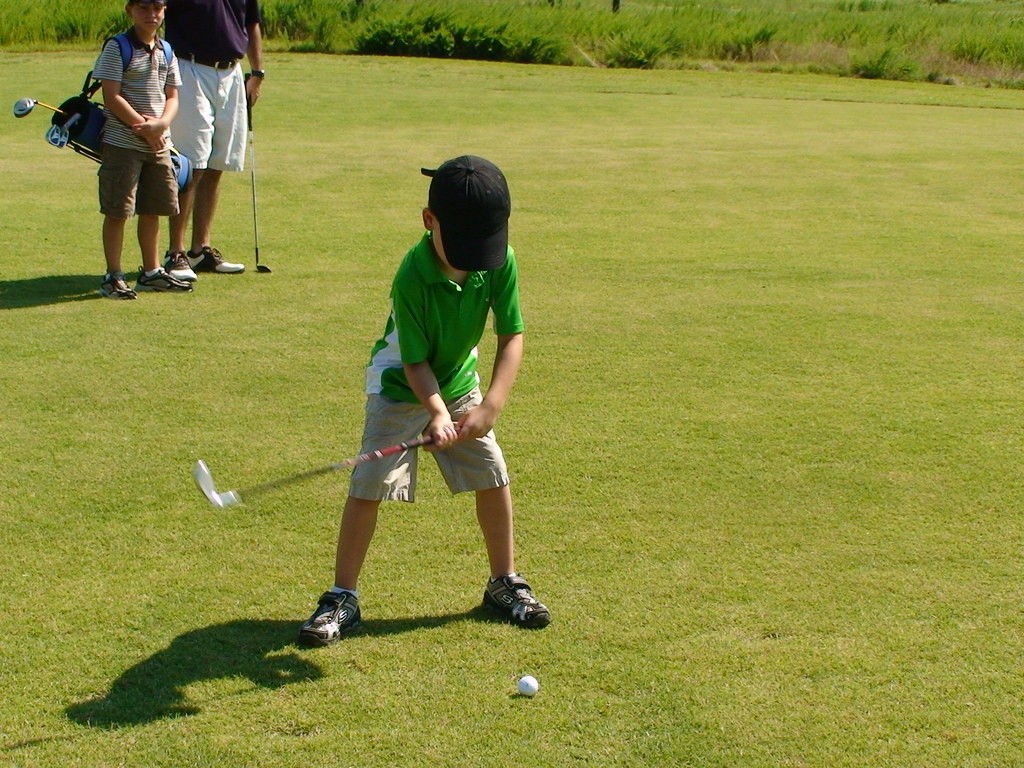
[427,155,511,272]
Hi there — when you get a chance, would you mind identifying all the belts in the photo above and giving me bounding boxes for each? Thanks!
[173,48,240,70]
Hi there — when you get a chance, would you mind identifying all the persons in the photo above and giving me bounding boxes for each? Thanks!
[92,0,192,301]
[161,0,265,281]
[297,155,552,646]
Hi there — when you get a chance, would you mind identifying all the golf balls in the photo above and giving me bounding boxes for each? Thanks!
[518,675,539,697]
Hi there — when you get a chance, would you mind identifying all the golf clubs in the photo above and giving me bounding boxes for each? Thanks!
[13,96,82,149]
[243,72,270,273]
[197,424,461,510]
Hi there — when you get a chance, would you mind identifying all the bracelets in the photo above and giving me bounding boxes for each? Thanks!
[251,70,264,79]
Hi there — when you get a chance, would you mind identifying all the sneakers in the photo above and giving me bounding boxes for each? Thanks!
[163,250,198,282]
[187,246,244,274]
[100,272,138,300]
[482,572,553,629]
[300,590,360,646]
[135,266,192,293]
